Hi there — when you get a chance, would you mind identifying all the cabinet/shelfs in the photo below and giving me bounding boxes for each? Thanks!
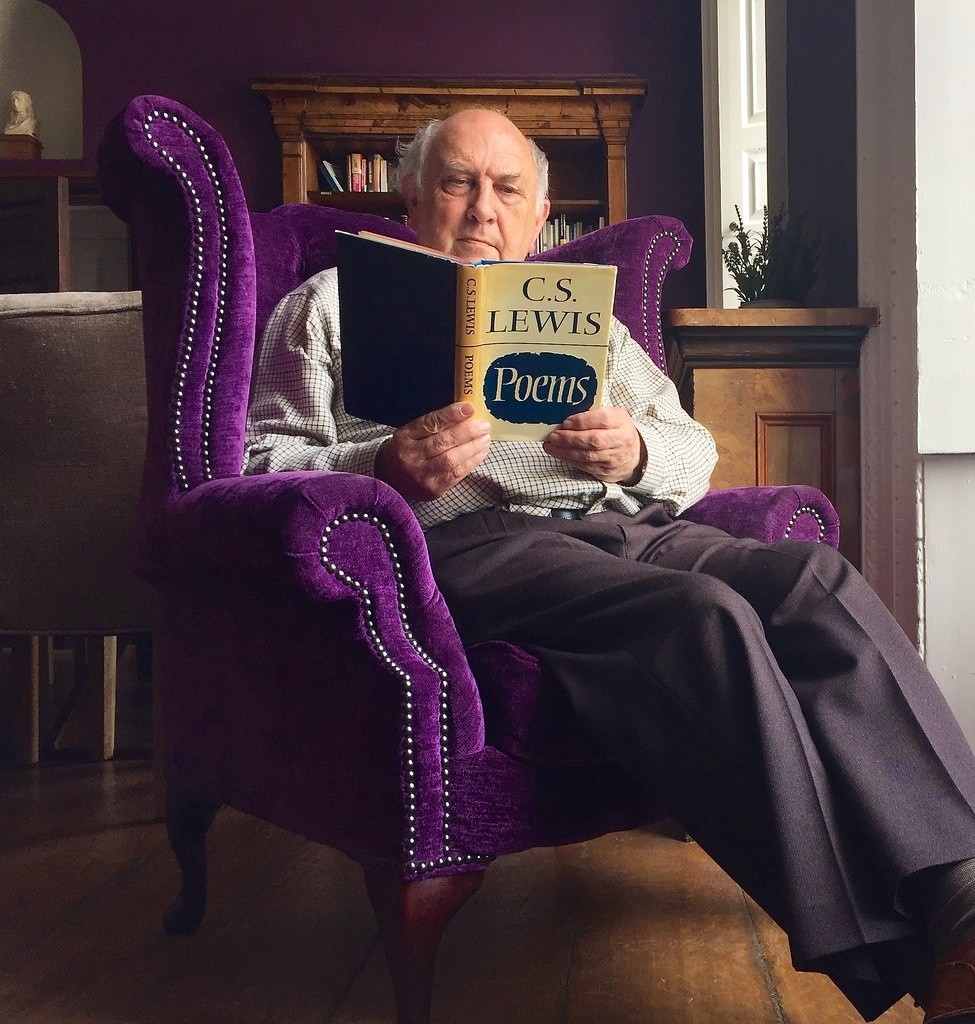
[249,78,645,257]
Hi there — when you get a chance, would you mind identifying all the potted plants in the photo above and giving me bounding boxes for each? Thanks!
[721,202,826,310]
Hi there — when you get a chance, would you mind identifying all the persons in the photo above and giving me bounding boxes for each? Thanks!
[239,108,975,1024]
[3,90,38,136]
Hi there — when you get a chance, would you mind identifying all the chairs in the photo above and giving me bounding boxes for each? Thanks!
[108,91,839,1023]
[0,289,152,765]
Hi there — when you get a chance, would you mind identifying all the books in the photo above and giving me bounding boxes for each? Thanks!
[334,230,618,444]
[526,214,604,258]
[317,151,400,193]
